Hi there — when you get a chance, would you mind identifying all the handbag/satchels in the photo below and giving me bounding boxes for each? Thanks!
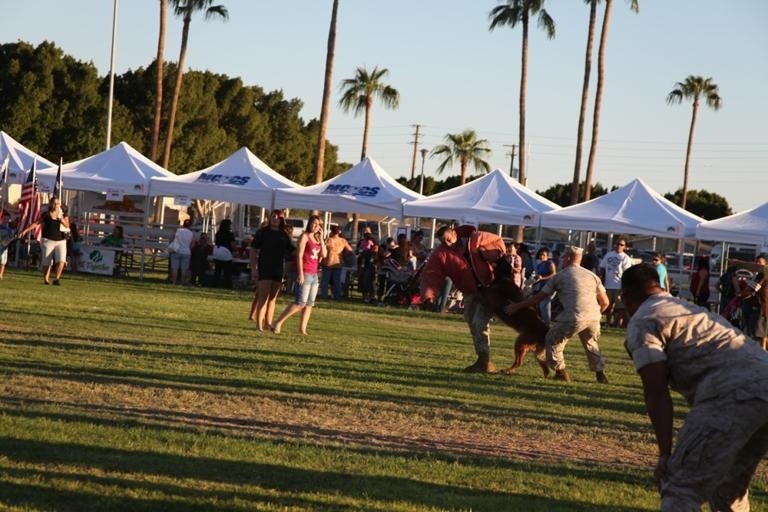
[167,239,180,254]
[344,252,356,267]
[59,222,71,232]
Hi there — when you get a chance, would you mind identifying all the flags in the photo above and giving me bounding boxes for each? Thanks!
[17,161,43,242]
[53,166,66,207]
[0,155,11,222]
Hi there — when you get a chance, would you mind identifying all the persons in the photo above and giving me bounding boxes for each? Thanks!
[690,254,767,352]
[620,263,766,511]
[504,244,612,383]
[165,207,432,337]
[61,204,70,226]
[0,209,13,280]
[502,239,671,325]
[418,228,519,373]
[18,197,70,285]
[101,225,124,261]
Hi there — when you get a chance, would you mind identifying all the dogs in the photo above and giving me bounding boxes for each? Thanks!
[475,248,551,378]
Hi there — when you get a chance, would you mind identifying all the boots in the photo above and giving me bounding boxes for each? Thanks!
[553,369,570,382]
[463,353,496,373]
[596,370,609,384]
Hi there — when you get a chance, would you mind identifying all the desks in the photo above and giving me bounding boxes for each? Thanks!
[33,240,134,280]
[342,265,358,299]
[207,255,251,275]
